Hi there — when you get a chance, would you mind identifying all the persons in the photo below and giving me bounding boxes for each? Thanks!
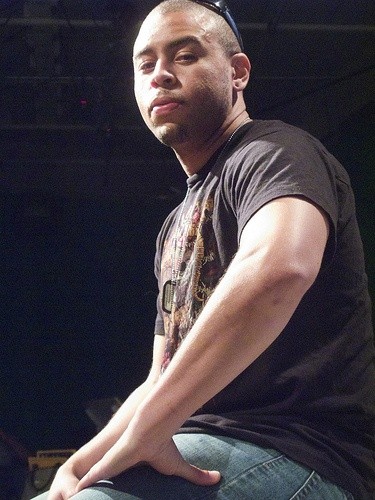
[23,0,375,500]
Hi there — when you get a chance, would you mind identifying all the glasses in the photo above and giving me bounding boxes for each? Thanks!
[192,0,244,53]
